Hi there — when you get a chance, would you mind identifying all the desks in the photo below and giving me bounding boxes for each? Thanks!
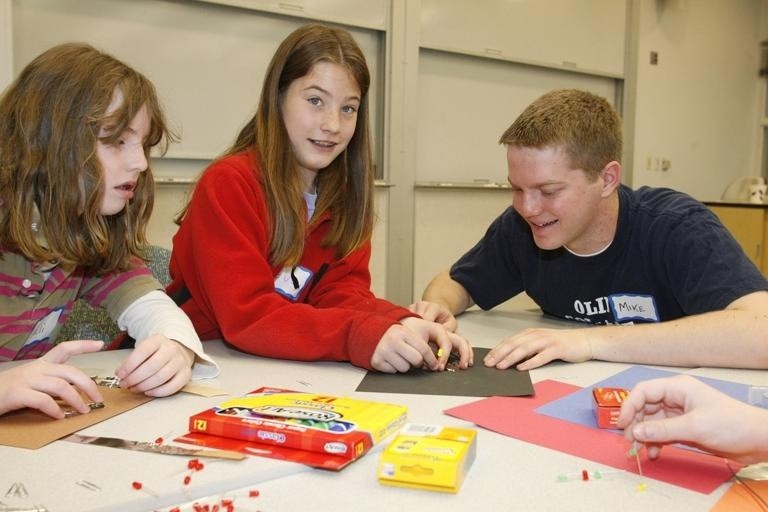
[0,310,767,512]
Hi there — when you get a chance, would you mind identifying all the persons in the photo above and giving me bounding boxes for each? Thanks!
[107,23,476,376]
[615,374,768,467]
[1,42,222,420]
[405,88,768,371]
